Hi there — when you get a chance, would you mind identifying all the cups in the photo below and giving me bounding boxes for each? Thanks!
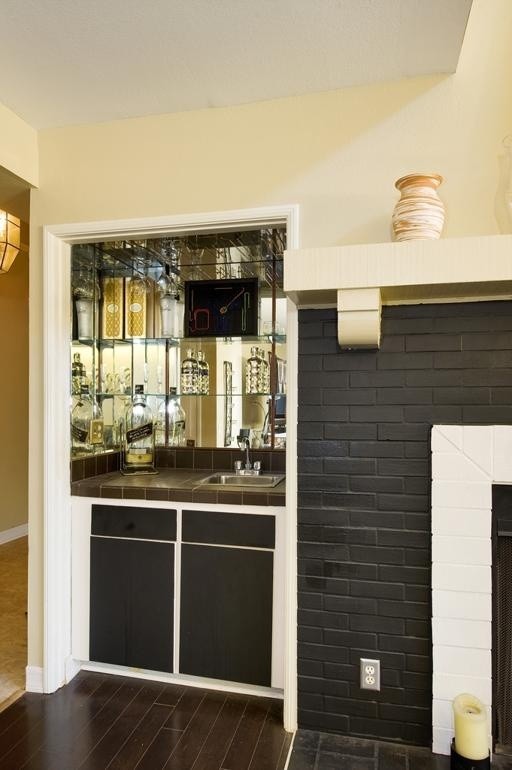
[76,299,97,341]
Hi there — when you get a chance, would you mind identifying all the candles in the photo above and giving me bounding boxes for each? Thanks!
[452,692,491,762]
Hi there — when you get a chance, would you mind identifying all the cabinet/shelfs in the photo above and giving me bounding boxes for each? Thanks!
[73,502,284,699]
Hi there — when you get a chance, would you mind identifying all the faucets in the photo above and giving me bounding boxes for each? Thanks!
[239,436,254,469]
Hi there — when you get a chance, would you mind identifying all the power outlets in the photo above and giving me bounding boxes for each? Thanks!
[359,657,381,692]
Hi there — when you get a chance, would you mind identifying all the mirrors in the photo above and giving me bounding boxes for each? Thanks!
[71,225,286,463]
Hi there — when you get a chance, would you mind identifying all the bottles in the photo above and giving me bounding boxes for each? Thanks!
[197,351,210,396]
[154,386,187,448]
[120,384,158,474]
[261,349,269,392]
[70,385,104,458]
[245,347,263,395]
[180,349,200,394]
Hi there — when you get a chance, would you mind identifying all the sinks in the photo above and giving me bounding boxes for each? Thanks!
[193,472,285,488]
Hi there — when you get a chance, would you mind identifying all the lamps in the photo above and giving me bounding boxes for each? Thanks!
[0,209,21,274]
